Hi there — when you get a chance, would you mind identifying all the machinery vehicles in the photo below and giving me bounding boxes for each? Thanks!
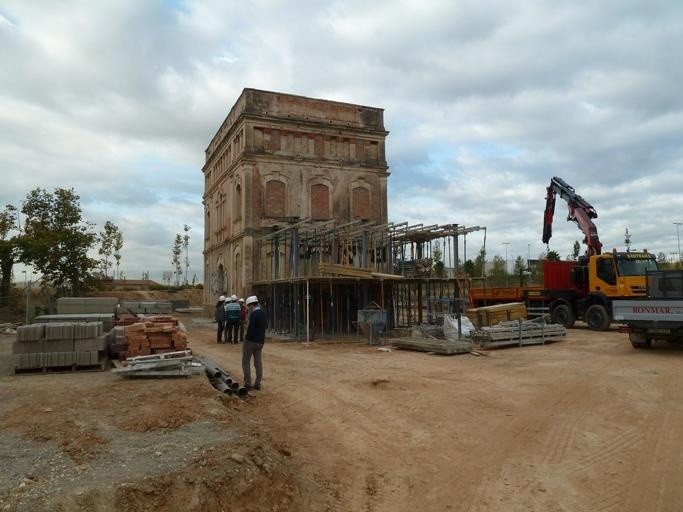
[468,176,658,331]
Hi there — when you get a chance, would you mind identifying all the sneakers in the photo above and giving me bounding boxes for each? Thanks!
[244,383,262,392]
[216,339,244,345]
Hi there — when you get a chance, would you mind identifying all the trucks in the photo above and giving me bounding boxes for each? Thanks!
[613,269,683,348]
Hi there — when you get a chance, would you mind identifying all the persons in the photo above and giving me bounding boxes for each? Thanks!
[213,294,247,346]
[240,295,266,392]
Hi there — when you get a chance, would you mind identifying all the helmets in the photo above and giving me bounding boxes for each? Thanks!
[218,294,244,303]
[245,295,259,306]
